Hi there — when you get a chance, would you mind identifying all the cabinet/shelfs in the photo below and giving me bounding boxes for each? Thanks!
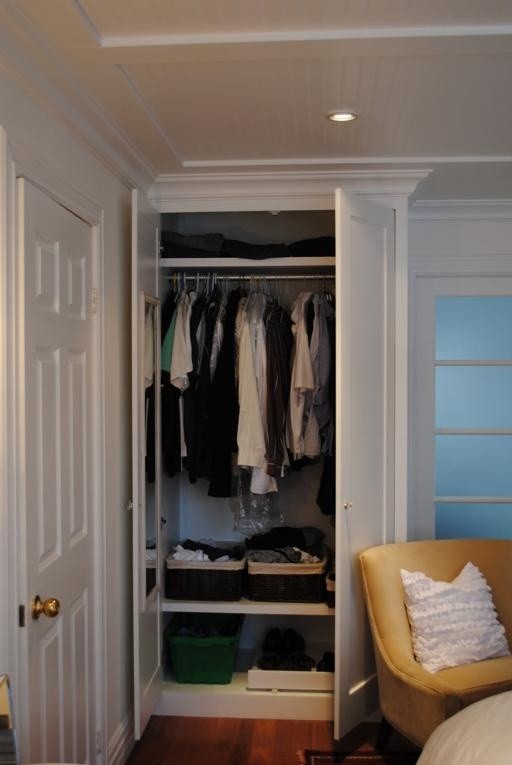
[129,160,430,742]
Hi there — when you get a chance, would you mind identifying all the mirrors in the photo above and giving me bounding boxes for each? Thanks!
[137,291,164,613]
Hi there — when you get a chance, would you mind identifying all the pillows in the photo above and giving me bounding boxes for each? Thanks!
[397,563,511,673]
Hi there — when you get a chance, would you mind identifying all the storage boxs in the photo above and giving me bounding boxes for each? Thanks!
[163,615,243,684]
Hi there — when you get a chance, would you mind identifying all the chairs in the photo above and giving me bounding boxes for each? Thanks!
[356,538,512,752]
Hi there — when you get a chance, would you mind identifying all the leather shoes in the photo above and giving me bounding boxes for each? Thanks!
[259,626,309,671]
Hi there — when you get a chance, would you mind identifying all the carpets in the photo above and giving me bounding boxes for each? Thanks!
[298,747,420,765]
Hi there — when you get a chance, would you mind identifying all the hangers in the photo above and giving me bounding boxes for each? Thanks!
[168,269,333,330]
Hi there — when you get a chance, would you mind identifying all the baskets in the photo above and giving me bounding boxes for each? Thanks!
[324,562,336,608]
[247,543,330,603]
[165,539,247,602]
